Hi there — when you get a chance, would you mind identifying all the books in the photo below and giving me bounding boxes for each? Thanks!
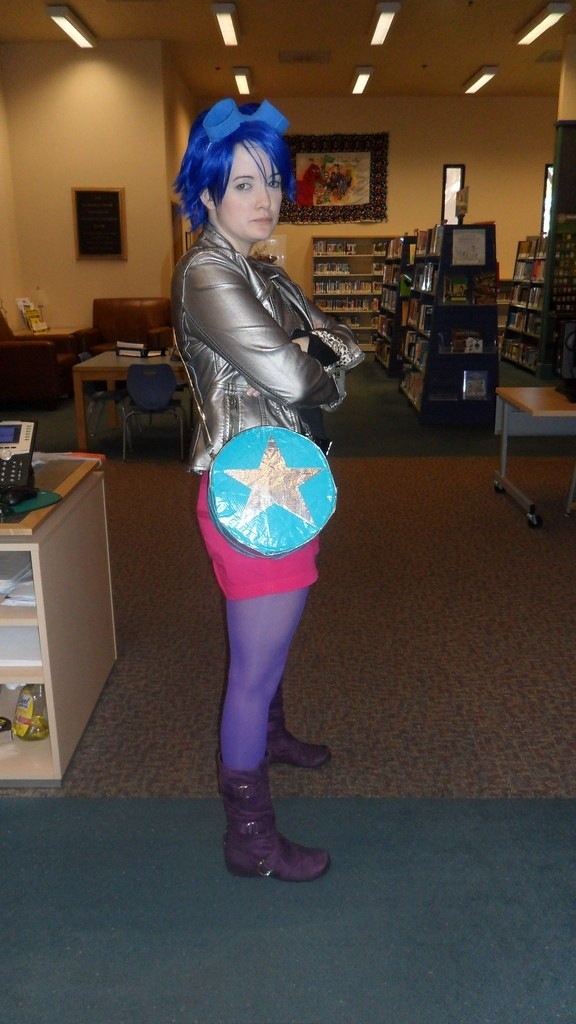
[315,281,371,294]
[386,238,404,261]
[372,262,385,275]
[315,262,351,275]
[381,288,397,312]
[383,263,402,286]
[400,298,434,406]
[371,298,381,312]
[377,315,392,341]
[371,333,378,344]
[374,340,391,366]
[372,282,382,294]
[410,225,446,294]
[336,315,360,327]
[372,241,387,255]
[371,316,378,329]
[313,240,356,255]
[502,238,546,370]
[315,299,369,312]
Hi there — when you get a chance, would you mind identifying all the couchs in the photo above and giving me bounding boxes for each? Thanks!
[0,310,76,412]
[73,297,174,394]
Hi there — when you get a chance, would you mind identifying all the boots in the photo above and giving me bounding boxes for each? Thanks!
[267,675,332,770]
[216,750,331,883]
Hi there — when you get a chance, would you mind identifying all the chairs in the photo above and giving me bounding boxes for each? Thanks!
[122,365,186,461]
[78,352,142,438]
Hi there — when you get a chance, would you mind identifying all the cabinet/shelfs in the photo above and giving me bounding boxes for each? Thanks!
[0,452,118,788]
[312,187,550,425]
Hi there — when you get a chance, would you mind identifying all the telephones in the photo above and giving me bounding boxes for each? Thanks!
[0,420,38,492]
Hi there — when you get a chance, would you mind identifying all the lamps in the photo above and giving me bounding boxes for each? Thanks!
[350,67,375,95]
[462,66,498,94]
[210,4,240,47]
[368,2,402,46]
[233,68,251,95]
[46,6,97,49]
[514,3,572,45]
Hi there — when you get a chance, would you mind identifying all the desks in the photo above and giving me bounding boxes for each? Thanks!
[491,387,576,531]
[72,351,201,451]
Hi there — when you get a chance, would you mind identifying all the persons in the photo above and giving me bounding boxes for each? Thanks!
[170,100,366,883]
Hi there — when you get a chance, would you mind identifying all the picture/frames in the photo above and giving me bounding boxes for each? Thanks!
[70,187,128,261]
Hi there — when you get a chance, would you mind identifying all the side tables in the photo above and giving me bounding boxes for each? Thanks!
[13,325,83,335]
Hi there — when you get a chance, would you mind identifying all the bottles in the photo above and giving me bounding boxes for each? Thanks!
[12,683,50,741]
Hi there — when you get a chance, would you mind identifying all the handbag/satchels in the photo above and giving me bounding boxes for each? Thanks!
[208,426,338,559]
